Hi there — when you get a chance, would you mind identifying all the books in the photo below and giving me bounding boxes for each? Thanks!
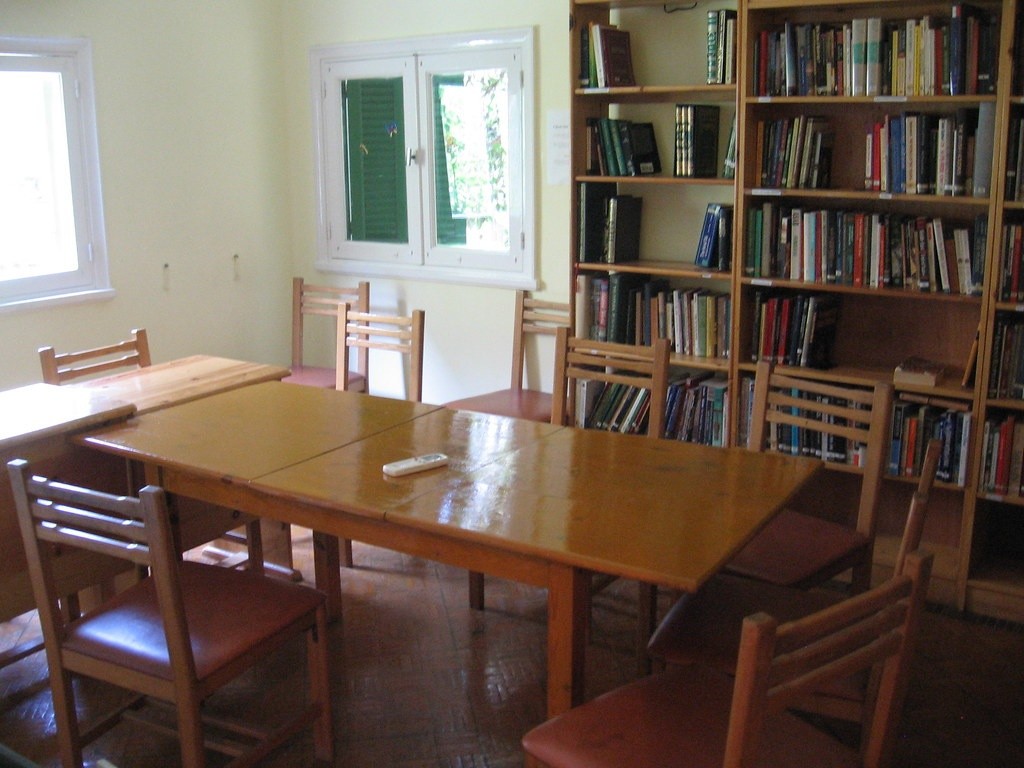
[572,371,729,448]
[576,182,734,273]
[751,5,1024,98]
[574,275,733,362]
[707,9,738,84]
[584,105,736,178]
[743,203,1024,498]
[578,22,638,88]
[753,101,1024,201]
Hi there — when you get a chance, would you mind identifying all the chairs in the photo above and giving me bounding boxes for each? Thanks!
[230,276,1023,768]
[5,457,335,768]
[36,327,151,384]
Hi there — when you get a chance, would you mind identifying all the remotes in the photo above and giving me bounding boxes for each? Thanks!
[383,454,448,477]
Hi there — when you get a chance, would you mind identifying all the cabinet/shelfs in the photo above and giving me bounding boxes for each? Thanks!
[566,2,1023,624]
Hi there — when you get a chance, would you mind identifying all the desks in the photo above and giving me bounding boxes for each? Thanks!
[0,355,292,691]
[69,378,825,721]
